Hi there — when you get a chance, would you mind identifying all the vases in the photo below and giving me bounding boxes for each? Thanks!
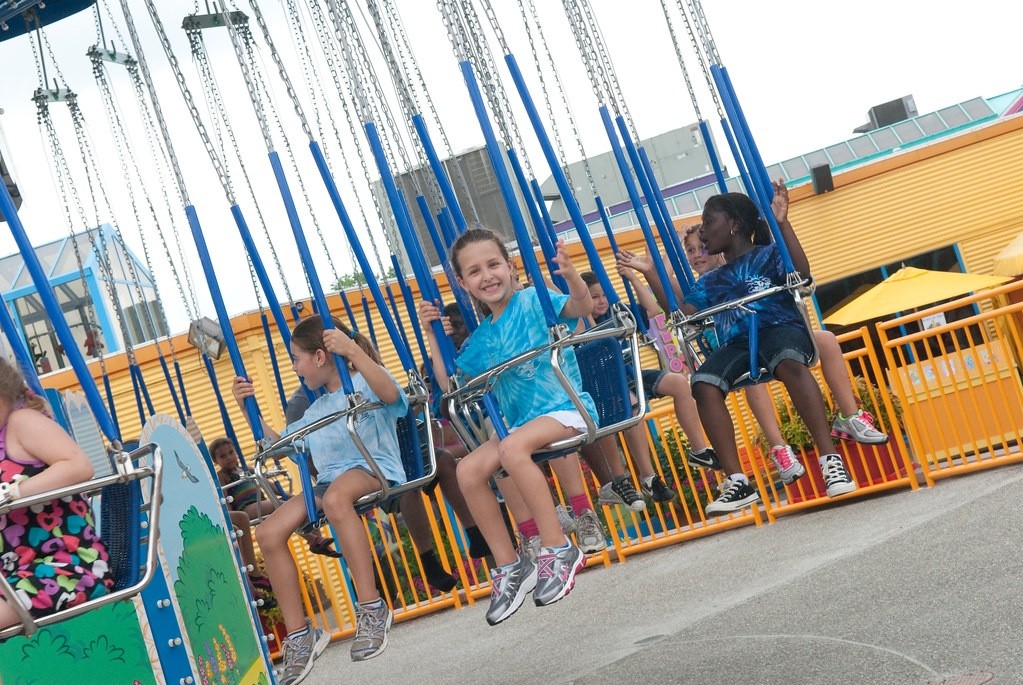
[676,510,709,527]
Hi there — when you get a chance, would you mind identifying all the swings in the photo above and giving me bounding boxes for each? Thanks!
[0,0,826,647]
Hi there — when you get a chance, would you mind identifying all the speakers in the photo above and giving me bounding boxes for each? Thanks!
[810,163,835,195]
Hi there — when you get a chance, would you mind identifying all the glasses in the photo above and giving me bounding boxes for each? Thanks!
[422,375,431,384]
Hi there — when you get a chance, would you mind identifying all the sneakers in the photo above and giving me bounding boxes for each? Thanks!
[830,409,889,445]
[515,532,542,564]
[350,598,394,661]
[640,473,676,501]
[279,619,331,685]
[820,455,855,498]
[705,475,759,517]
[599,482,644,503]
[555,505,577,535]
[485,553,538,626]
[769,443,806,485]
[533,526,587,606]
[610,478,646,511]
[688,447,724,470]
[574,507,609,553]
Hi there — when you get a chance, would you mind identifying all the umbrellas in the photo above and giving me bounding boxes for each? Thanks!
[822,233,1023,334]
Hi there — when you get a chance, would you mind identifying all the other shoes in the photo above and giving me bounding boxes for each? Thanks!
[249,576,272,590]
[313,547,342,558]
[309,536,334,550]
[254,591,277,609]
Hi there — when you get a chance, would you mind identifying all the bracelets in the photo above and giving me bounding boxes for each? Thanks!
[10,480,21,500]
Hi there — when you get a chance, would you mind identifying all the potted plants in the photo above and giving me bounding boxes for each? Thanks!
[750,374,918,505]
[255,551,315,648]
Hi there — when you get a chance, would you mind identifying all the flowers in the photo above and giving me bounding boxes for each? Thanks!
[393,526,488,606]
[625,426,722,520]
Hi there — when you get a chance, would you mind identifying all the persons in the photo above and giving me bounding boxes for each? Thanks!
[0,356,115,629]
[232,314,410,685]
[420,358,433,392]
[229,511,278,610]
[662,223,889,485]
[442,303,469,349]
[615,177,856,518]
[285,385,492,592]
[209,438,343,558]
[580,271,725,503]
[417,229,595,626]
[490,262,608,562]
